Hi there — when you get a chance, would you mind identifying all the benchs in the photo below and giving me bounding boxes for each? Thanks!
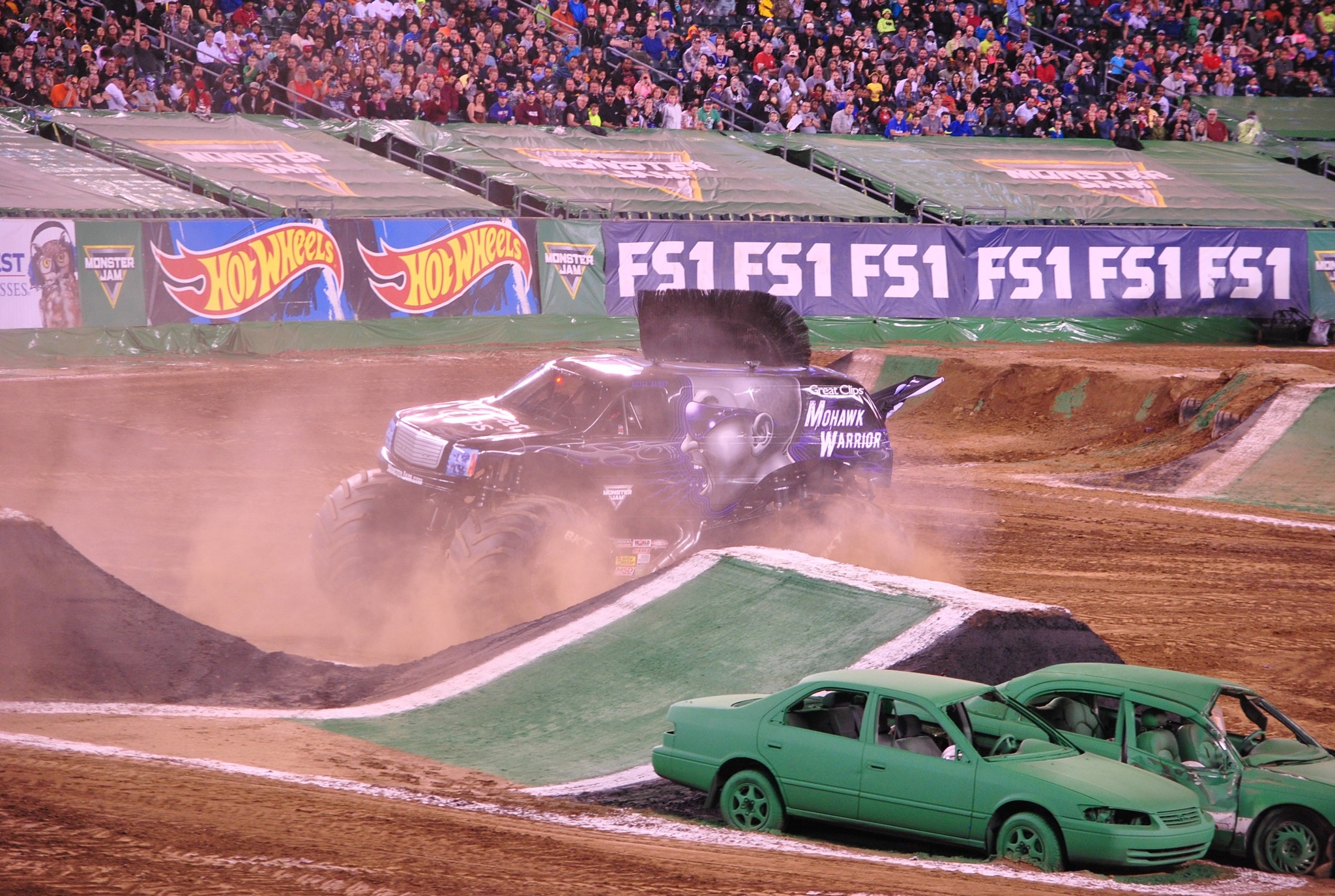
[1027,696,1114,740]
[787,691,864,740]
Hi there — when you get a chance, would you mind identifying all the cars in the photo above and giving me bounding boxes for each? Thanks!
[891,660,1335,879]
[650,668,1217,875]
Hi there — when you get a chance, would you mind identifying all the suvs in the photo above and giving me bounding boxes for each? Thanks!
[310,286,891,635]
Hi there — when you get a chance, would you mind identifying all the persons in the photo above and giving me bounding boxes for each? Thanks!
[0,0,1335,147]
[678,373,872,565]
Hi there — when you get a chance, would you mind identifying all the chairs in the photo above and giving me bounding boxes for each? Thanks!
[1136,708,1181,766]
[895,714,942,757]
[1176,716,1223,769]
